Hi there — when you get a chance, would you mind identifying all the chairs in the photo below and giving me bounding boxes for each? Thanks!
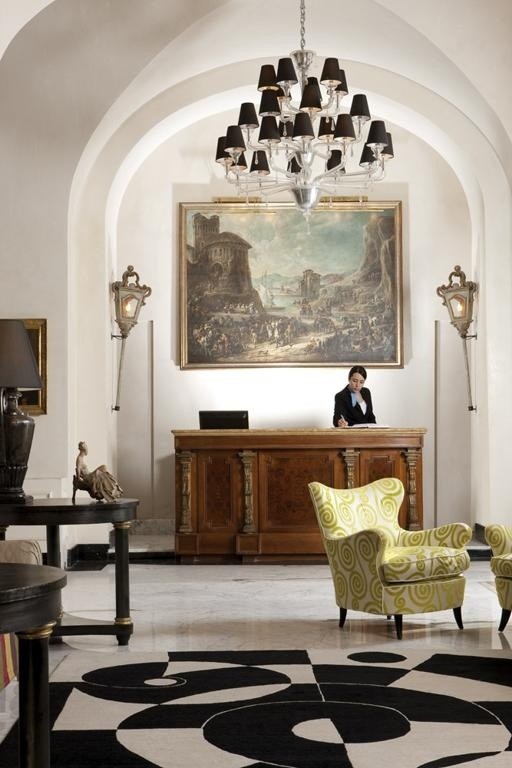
[0,536,49,688]
[485,522,512,632]
[307,476,474,640]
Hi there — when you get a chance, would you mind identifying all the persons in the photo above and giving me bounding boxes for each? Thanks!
[333,366,376,427]
[187,277,396,357]
[76,441,124,505]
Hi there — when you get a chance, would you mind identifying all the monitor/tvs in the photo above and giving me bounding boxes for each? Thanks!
[199,411,249,430]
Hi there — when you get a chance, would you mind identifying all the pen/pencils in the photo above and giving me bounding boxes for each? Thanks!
[340,414,346,422]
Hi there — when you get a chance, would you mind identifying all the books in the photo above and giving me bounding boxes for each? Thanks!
[343,424,389,429]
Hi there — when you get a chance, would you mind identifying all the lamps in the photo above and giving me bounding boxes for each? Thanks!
[0,319,44,504]
[109,264,153,411]
[215,0,395,235]
[436,264,479,411]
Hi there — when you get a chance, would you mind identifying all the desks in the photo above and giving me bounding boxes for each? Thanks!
[0,497,140,646]
[1,561,68,767]
[170,426,426,567]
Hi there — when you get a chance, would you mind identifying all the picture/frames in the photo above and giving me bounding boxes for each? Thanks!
[17,319,47,416]
[178,201,405,371]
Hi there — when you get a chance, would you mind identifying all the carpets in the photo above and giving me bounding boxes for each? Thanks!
[0,648,512,768]
